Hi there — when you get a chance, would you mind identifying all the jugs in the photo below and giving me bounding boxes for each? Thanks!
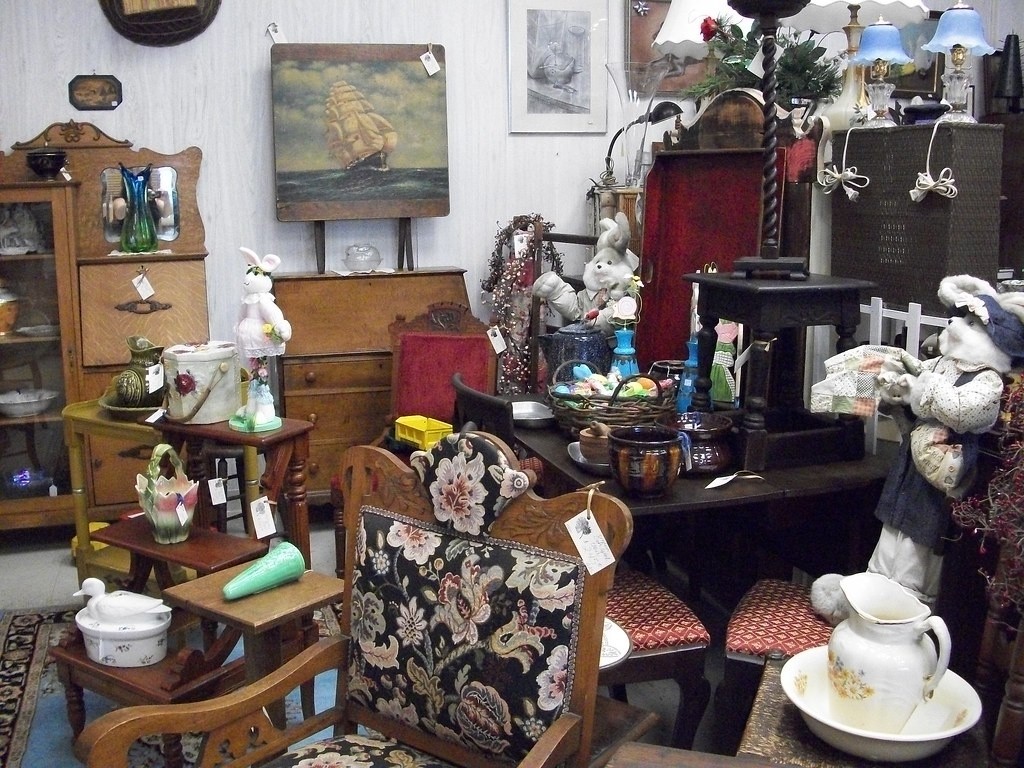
[829,572,951,734]
[117,335,165,407]
[538,310,617,385]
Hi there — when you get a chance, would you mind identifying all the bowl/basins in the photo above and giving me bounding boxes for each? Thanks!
[26,141,67,181]
[780,645,982,761]
[0,389,58,417]
[654,412,733,475]
[580,425,625,463]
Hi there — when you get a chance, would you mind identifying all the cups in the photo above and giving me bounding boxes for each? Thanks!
[608,426,683,499]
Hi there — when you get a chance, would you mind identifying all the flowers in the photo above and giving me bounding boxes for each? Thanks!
[675,12,859,103]
[263,323,290,343]
[633,1,651,17]
[478,212,564,399]
[250,356,268,386]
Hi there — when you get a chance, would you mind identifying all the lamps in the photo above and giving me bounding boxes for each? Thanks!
[780,0,930,129]
[921,0,996,124]
[596,100,684,191]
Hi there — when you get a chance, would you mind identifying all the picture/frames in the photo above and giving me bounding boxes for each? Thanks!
[983,50,1010,116]
[865,10,945,101]
[68,75,123,111]
[625,0,717,98]
[942,85,975,118]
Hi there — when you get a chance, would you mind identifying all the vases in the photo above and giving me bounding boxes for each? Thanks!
[708,77,834,119]
[116,161,158,252]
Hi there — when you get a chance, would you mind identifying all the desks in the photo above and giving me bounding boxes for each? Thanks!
[163,559,345,768]
[734,648,990,768]
[492,393,900,708]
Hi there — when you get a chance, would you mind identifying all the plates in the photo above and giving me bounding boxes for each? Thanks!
[98,396,159,418]
[596,616,632,672]
[511,401,554,426]
[18,325,59,333]
[567,441,609,477]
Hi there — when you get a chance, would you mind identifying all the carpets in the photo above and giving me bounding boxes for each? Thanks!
[0,600,388,768]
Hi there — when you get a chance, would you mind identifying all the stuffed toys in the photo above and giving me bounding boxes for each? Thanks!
[533,212,638,349]
[811,275,1024,624]
[235,247,291,358]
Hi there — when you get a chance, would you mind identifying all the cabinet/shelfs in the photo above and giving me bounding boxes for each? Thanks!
[271,266,472,530]
[61,397,261,609]
[48,412,319,768]
[979,114,1024,279]
[0,119,209,533]
[829,122,1006,319]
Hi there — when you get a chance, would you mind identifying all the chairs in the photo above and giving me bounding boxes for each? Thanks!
[329,299,510,579]
[710,460,980,756]
[452,371,712,751]
[73,433,634,768]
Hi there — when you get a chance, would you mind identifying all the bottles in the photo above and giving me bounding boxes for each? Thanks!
[118,162,159,253]
[0,286,19,332]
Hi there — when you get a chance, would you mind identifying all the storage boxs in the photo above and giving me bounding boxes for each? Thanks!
[394,415,453,451]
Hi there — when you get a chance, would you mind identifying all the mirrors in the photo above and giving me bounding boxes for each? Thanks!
[100,167,181,243]
